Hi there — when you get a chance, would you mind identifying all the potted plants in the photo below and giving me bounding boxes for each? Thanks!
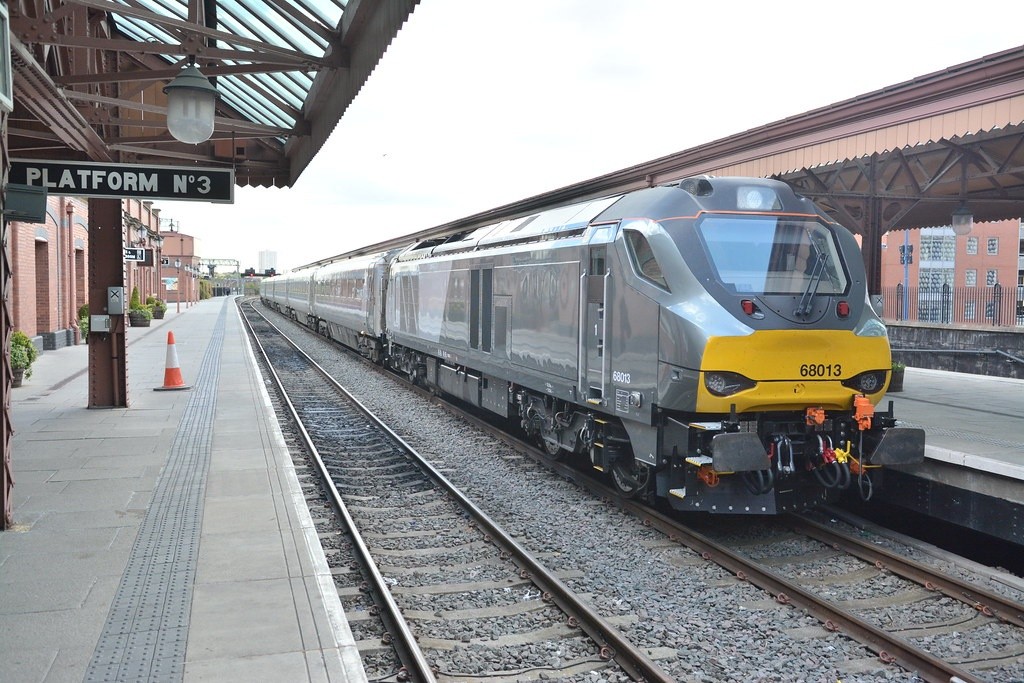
[127,305,152,327]
[80,318,88,344]
[9,343,29,387]
[154,305,165,319]
[886,362,905,391]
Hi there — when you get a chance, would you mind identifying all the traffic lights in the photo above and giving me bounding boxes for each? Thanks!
[900,256,905,264]
[907,254,912,264]
[907,244,913,253]
[899,245,905,254]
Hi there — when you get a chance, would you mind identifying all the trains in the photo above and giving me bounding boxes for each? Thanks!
[258,173,926,517]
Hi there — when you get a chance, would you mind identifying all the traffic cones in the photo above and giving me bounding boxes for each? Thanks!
[152,330,194,391]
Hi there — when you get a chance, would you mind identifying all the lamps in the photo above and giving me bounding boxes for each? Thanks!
[161,55,222,145]
[951,203,973,235]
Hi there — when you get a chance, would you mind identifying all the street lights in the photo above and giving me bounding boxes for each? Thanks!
[220,275,224,296]
[208,274,212,295]
[214,275,217,297]
[190,265,193,307]
[184,263,190,309]
[193,267,197,305]
[174,258,182,314]
[196,269,201,303]
